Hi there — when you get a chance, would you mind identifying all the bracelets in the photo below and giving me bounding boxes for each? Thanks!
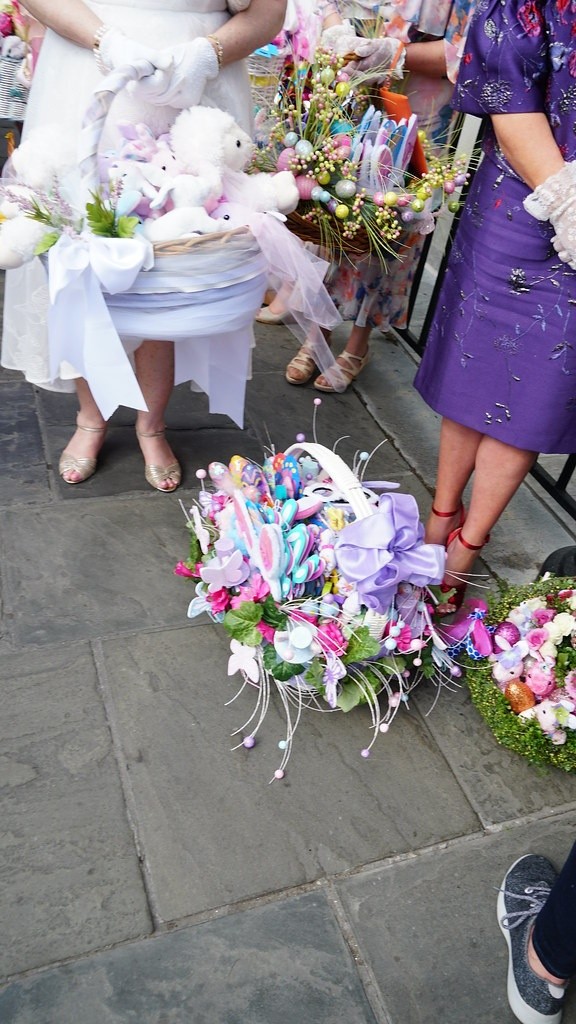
[207,34,224,69]
[93,24,110,47]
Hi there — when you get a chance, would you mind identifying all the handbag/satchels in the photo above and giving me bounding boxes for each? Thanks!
[379,42,429,181]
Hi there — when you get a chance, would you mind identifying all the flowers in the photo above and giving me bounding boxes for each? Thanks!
[173,397,576,785]
[0,171,141,257]
[244,31,484,274]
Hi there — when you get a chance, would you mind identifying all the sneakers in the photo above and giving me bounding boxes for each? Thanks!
[497,854,570,1024]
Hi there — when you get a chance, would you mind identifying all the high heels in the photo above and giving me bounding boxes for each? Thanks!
[255,300,304,324]
[434,527,491,618]
[432,497,466,531]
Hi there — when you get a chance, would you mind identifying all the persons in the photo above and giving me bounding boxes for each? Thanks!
[0,0,576,615]
[493,841,576,1024]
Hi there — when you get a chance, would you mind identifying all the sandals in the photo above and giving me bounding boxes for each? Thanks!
[58,425,107,484]
[285,331,332,384]
[314,344,370,392]
[135,425,182,492]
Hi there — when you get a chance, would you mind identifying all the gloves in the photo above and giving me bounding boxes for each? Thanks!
[148,37,219,109]
[93,25,174,98]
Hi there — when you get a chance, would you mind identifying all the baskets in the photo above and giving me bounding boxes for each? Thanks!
[251,96,420,255]
[256,441,388,692]
[464,575,576,773]
[0,55,30,120]
[243,44,292,125]
